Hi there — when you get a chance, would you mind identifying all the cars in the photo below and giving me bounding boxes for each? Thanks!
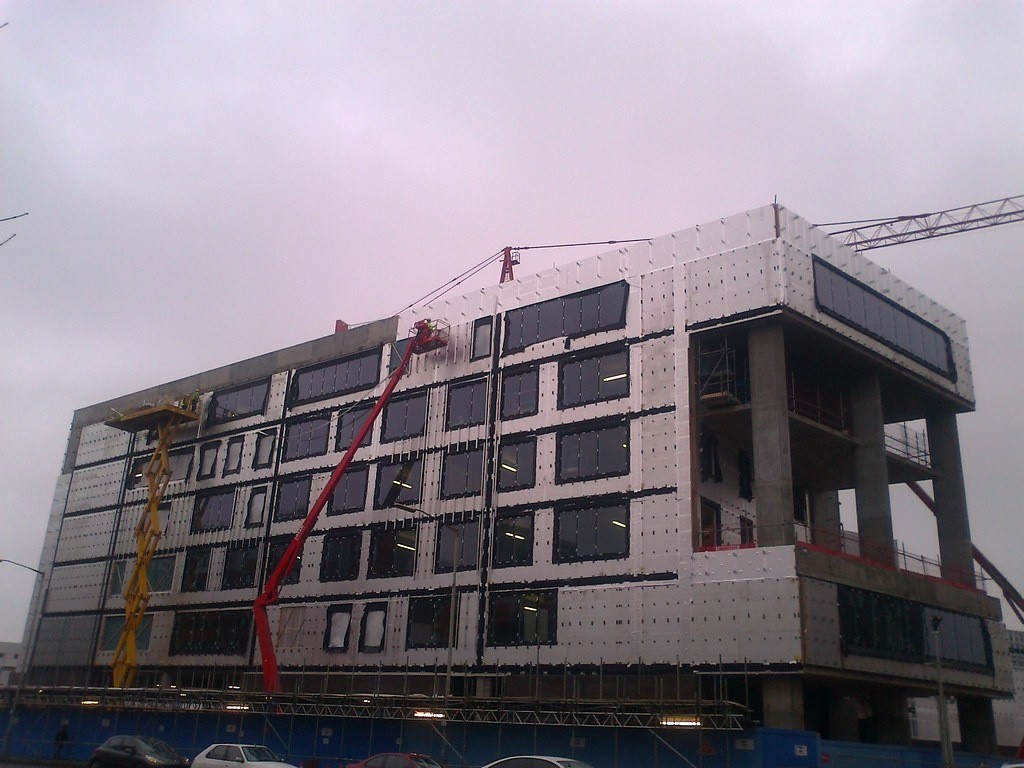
[345,754,444,768]
[193,743,295,768]
[481,755,593,768]
[91,735,191,768]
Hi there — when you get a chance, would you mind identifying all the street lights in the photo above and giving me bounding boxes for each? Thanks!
[397,502,459,727]
[1,558,47,762]
[931,612,955,767]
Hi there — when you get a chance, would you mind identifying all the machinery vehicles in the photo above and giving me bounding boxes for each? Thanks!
[102,393,202,707]
[252,316,453,712]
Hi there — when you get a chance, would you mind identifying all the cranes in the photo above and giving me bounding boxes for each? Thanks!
[336,191,1024,332]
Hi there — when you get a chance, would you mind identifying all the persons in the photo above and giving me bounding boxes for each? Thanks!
[182,397,187,410]
[191,388,201,412]
[53,724,68,760]
[426,318,437,338]
